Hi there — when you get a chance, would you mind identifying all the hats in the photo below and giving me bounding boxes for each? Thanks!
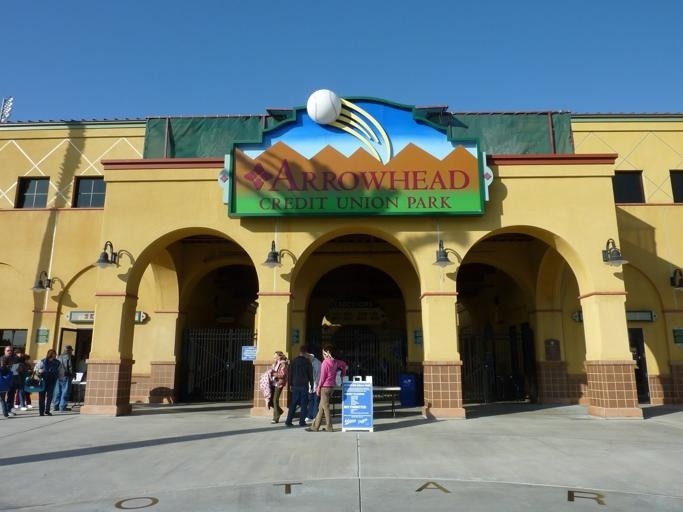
[64,346,72,350]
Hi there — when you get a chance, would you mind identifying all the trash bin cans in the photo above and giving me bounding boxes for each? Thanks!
[397,372,419,407]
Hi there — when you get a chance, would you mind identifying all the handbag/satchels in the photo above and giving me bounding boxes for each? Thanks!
[24,377,45,393]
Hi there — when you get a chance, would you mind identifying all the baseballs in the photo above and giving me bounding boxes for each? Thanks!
[307,89,341,124]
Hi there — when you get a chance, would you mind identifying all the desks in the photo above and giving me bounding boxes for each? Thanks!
[332,386,402,418]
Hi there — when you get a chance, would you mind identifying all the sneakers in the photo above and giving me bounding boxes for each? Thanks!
[53,408,71,411]
[284,416,334,432]
[271,409,282,423]
[7,404,33,417]
[40,411,52,416]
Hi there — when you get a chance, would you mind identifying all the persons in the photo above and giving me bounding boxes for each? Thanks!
[272,343,342,432]
[0,345,87,418]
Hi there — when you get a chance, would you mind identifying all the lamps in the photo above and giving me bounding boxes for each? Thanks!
[261,239,282,267]
[432,239,451,268]
[602,239,629,267]
[670,268,683,290]
[30,241,118,293]
